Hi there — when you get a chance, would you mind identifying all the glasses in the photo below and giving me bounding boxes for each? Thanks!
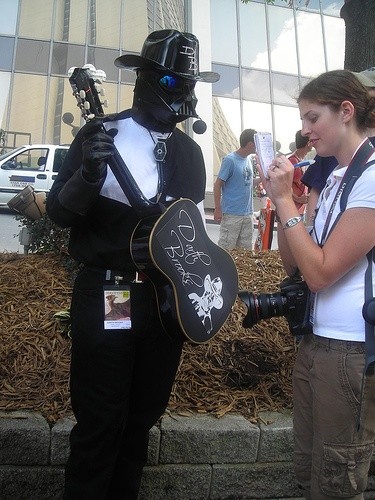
[150,76,195,94]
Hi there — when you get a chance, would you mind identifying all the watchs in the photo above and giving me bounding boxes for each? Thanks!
[282,216,303,229]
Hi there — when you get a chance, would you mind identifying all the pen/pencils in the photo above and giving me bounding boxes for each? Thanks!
[293,159,316,169]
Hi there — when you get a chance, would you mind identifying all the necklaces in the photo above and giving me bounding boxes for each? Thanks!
[147,128,172,162]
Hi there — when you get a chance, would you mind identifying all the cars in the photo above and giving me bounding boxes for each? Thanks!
[0,143,74,213]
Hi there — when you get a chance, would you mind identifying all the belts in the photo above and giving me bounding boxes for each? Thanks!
[80,265,149,284]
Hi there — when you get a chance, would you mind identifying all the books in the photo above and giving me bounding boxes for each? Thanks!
[253,132,275,181]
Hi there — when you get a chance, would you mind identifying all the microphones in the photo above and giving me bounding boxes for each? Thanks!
[193,116,207,134]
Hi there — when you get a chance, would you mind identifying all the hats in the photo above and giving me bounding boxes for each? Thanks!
[114,29,220,83]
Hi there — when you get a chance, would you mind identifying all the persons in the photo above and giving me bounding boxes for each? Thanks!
[254,68,375,500]
[45,29,208,500]
[300,85,375,224]
[212,128,257,250]
[288,129,313,227]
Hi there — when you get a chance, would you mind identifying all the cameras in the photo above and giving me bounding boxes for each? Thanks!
[237,274,313,336]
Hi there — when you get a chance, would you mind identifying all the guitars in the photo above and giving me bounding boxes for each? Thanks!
[67,64,238,345]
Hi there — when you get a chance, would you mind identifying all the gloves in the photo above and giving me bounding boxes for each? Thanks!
[58,128,119,214]
